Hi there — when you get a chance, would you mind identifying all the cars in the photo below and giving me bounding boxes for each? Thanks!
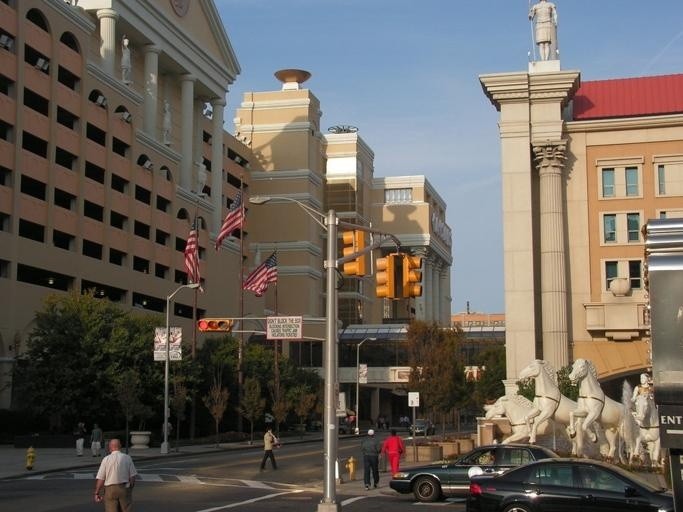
[408,418,435,435]
[466,458,675,511]
[389,444,562,503]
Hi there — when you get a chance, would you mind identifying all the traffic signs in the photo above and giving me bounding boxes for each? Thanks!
[266,314,302,340]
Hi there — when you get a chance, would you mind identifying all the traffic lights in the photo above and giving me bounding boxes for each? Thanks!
[343,229,364,277]
[402,253,422,299]
[376,252,400,301]
[198,319,233,332]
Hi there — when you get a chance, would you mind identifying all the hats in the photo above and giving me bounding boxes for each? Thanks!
[368,429,374,435]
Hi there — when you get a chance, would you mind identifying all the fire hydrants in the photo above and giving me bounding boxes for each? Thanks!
[25,446,35,470]
[345,455,356,481]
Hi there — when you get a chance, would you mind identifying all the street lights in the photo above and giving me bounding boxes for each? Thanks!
[158,282,202,455]
[247,195,337,511]
[354,337,377,435]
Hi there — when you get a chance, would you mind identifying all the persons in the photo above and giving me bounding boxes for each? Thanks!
[73,422,88,456]
[631,373,654,403]
[90,422,103,457]
[360,429,382,490]
[381,430,405,476]
[162,422,174,442]
[260,426,280,471]
[94,439,137,512]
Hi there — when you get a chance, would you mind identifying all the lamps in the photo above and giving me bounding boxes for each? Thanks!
[142,159,154,170]
[34,56,52,71]
[120,111,132,123]
[93,94,107,107]
[0,34,14,50]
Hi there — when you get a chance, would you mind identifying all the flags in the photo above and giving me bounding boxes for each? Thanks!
[216,188,244,253]
[243,251,278,296]
[184,212,204,294]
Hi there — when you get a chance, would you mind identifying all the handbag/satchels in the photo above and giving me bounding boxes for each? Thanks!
[399,448,403,454]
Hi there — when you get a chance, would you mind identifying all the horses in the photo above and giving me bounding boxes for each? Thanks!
[485,394,577,456]
[631,391,668,468]
[568,358,645,465]
[518,359,600,456]
